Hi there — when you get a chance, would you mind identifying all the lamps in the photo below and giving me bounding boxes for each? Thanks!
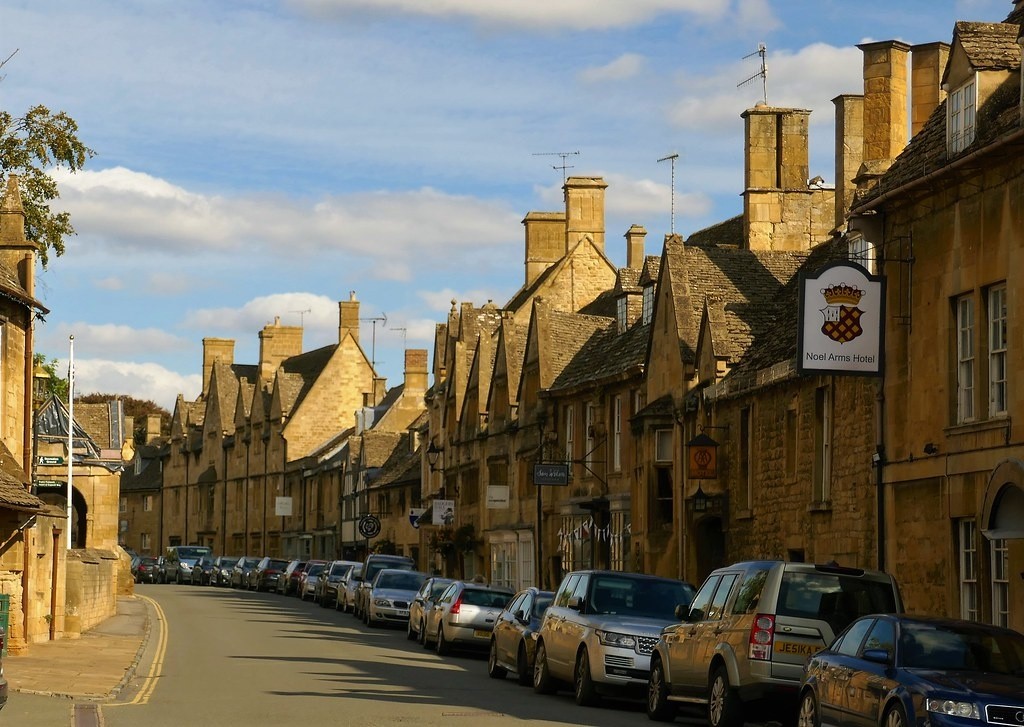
[426,438,445,474]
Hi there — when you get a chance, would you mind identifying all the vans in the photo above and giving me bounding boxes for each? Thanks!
[352,554,416,617]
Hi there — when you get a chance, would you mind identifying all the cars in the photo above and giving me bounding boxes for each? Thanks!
[313,562,333,602]
[248,557,291,591]
[335,565,363,613]
[488,585,557,678]
[533,569,699,705]
[407,577,458,640]
[228,556,262,588]
[423,581,517,656]
[301,564,325,600]
[296,560,327,597]
[363,568,427,628]
[128,546,239,587]
[275,558,307,596]
[321,560,363,608]
[798,613,1024,727]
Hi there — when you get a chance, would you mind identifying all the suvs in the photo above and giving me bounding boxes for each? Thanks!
[647,558,908,727]
